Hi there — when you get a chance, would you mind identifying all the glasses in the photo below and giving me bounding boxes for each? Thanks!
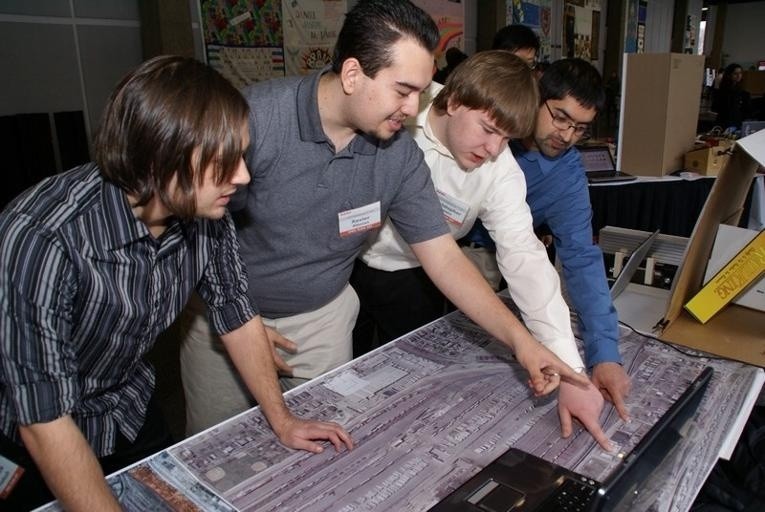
[543,99,593,140]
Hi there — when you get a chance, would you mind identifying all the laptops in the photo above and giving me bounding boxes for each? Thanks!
[428,366,715,512]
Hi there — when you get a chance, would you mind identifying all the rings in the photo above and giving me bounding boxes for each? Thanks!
[549,373,559,377]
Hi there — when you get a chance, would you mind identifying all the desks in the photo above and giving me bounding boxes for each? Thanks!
[30,275,765,511]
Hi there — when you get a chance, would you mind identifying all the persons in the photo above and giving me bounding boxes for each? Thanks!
[712,67,726,92]
[0,53,358,512]
[444,56,634,423]
[712,63,754,127]
[490,23,541,73]
[349,49,615,452]
[603,70,620,100]
[169,0,591,439]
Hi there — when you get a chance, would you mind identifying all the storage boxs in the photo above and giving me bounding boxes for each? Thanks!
[620,54,706,176]
[684,142,733,177]
